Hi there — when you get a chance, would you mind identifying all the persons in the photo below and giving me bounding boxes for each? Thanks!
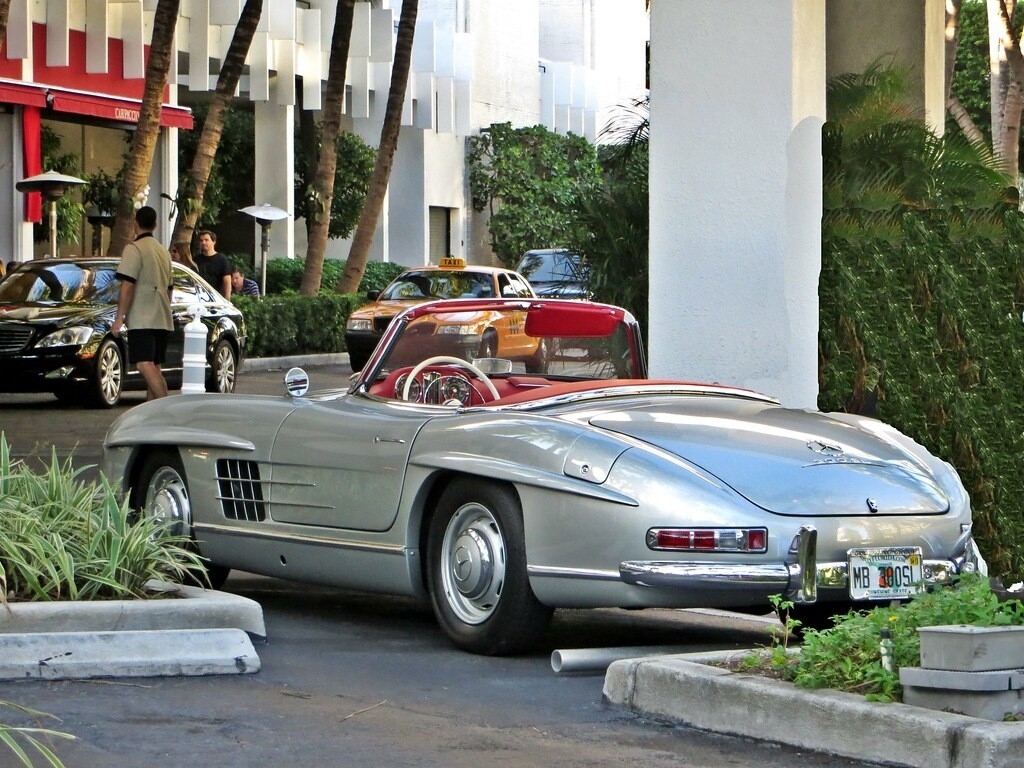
[193,231,231,301]
[231,268,259,296]
[110,206,174,401]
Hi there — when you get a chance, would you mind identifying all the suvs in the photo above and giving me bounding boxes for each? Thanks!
[515,249,613,359]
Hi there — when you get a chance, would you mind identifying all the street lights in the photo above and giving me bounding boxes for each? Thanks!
[237,203,291,296]
[15,169,89,257]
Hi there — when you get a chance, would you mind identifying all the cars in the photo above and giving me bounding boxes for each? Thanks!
[346,267,553,375]
[103,292,991,658]
[0,257,245,407]
